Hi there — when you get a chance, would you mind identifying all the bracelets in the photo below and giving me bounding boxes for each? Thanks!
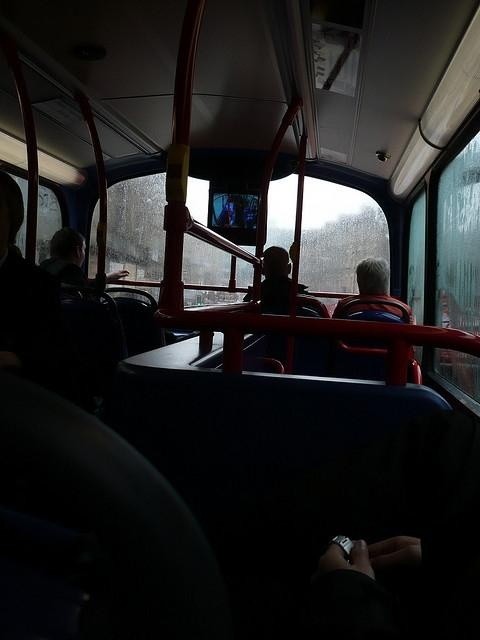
[327,532,356,556]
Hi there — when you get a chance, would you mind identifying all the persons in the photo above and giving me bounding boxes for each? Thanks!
[0,164,67,392]
[37,221,129,303]
[242,243,309,311]
[294,403,479,639]
[332,260,419,364]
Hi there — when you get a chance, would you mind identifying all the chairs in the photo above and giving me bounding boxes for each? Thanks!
[43,279,169,394]
[232,291,414,382]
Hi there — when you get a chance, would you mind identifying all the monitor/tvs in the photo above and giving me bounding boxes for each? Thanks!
[211,190,259,232]
